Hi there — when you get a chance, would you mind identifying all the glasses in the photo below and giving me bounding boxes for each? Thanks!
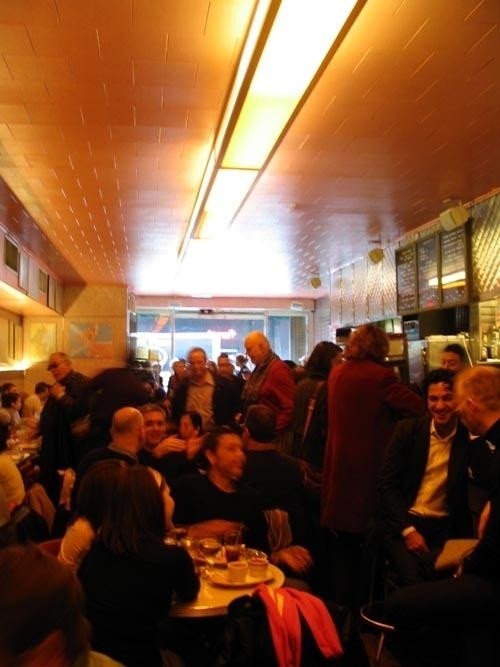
[48,362,58,370]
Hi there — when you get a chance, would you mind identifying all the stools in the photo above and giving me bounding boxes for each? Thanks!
[361,606,397,666]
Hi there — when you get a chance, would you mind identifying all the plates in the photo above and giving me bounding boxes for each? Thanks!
[212,572,275,587]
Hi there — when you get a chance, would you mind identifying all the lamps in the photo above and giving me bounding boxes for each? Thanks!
[180,0,369,266]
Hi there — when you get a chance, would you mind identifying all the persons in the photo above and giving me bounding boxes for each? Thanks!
[1,322,499,666]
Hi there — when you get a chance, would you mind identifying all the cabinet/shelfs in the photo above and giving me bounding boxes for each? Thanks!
[1,222,65,316]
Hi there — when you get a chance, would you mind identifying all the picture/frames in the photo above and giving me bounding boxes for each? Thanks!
[394,240,415,313]
[58,315,125,366]
[415,230,441,312]
[439,214,474,311]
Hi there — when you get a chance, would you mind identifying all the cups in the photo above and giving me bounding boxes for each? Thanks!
[248,558,269,577]
[225,531,241,561]
[198,538,221,554]
[227,561,248,580]
